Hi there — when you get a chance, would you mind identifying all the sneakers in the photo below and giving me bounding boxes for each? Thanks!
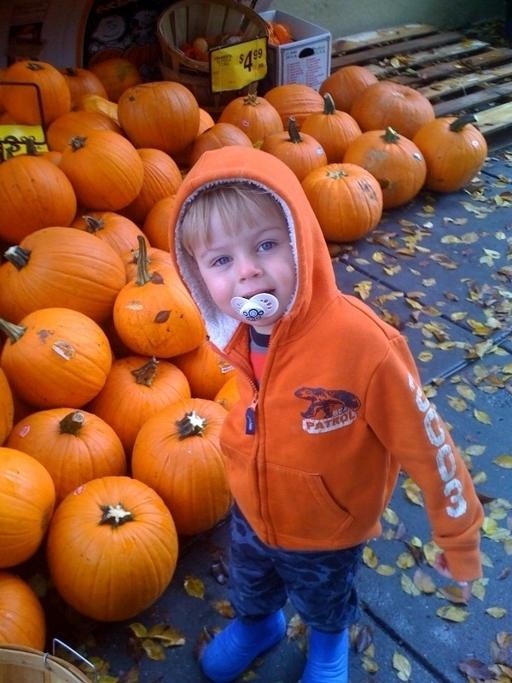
[201,609,287,683]
[301,626,349,683]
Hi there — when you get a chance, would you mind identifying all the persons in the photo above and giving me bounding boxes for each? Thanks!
[169,145,484,683]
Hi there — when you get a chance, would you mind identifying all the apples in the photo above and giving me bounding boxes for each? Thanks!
[180,37,209,62]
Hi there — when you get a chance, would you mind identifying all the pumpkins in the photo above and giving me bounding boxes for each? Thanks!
[1,47,487,653]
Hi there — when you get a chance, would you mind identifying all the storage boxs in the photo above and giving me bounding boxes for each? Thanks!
[255,6,333,102]
